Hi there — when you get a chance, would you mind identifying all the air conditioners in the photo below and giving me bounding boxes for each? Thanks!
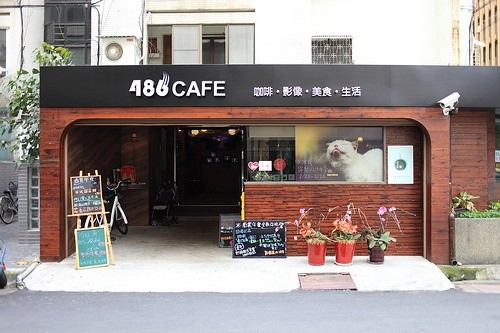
[100,37,137,65]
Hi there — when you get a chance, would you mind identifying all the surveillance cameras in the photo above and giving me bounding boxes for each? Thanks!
[438,91,461,108]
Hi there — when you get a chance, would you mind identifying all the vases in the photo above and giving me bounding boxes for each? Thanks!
[366,239,385,263]
[333,241,356,265]
[306,243,326,266]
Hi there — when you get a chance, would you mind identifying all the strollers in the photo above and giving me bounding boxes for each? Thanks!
[150,180,180,226]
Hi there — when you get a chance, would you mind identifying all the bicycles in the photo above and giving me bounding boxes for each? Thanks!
[0,181,18,225]
[83,178,128,244]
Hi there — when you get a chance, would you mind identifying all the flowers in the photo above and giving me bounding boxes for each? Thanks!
[284,202,416,251]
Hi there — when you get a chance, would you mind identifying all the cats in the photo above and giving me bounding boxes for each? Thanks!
[325,139,383,182]
